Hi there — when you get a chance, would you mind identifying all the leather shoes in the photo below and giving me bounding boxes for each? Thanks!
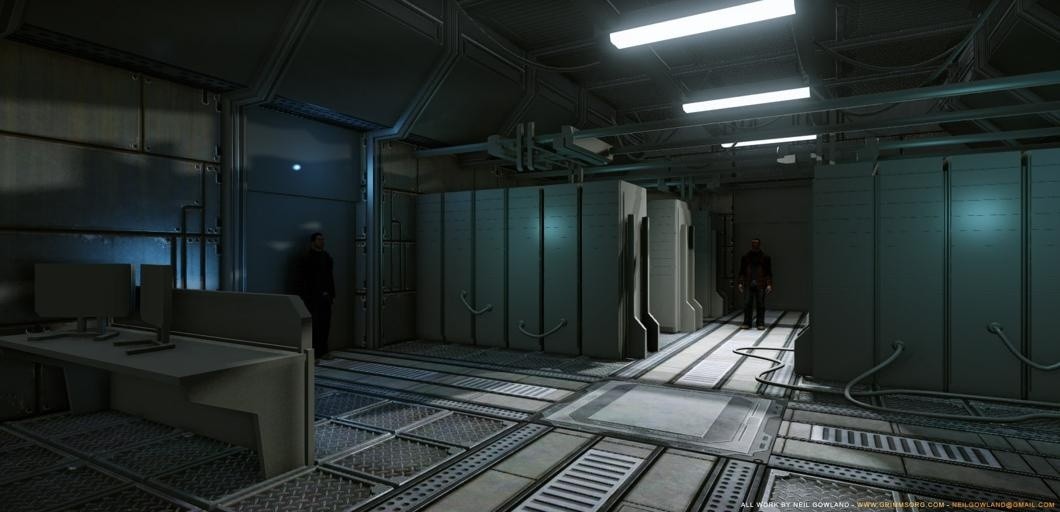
[315,353,334,364]
[741,323,765,330]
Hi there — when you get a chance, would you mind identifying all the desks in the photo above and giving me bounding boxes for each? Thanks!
[0,325,307,479]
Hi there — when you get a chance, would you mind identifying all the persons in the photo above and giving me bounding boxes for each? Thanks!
[306,232,336,363]
[738,239,773,330]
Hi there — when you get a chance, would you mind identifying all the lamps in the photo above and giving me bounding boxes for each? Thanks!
[609,0,798,52]
[683,76,812,114]
